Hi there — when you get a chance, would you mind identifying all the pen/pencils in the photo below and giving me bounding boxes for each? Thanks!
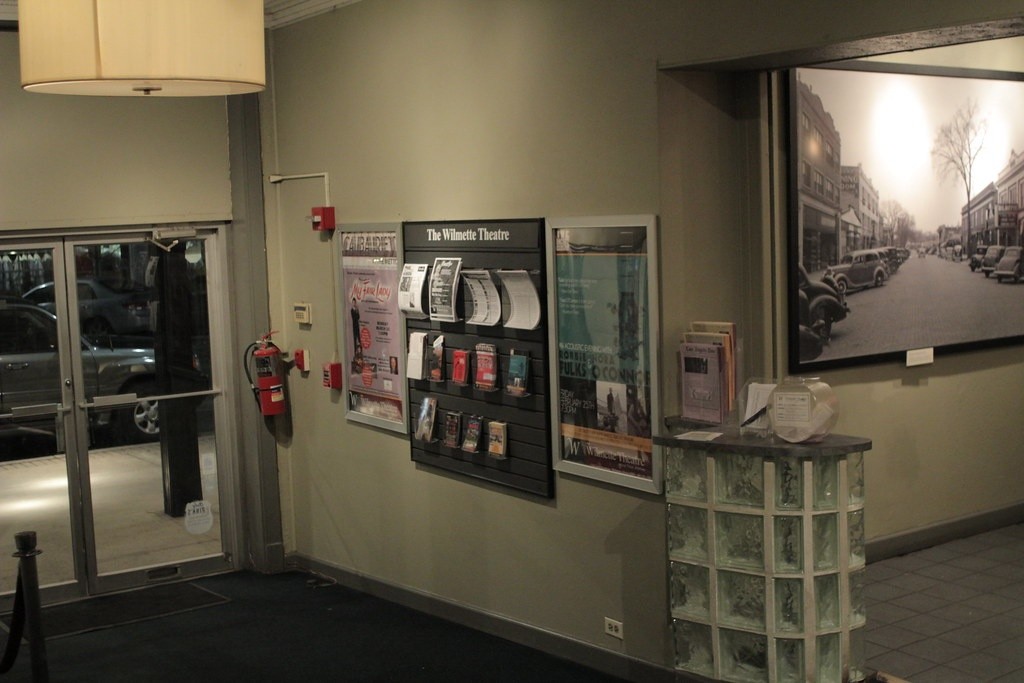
[740,404,766,427]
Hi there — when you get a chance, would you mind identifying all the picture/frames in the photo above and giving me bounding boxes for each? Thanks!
[787,59,1024,376]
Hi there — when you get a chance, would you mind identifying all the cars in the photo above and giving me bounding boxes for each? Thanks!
[994,246,1024,283]
[798,265,850,362]
[968,246,988,272]
[866,247,911,276]
[16,277,149,347]
[981,245,1006,277]
[0,297,208,440]
[820,249,888,296]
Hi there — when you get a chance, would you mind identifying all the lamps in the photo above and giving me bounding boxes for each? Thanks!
[17,0,267,97]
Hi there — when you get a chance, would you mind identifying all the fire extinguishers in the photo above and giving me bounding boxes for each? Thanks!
[243,330,286,416]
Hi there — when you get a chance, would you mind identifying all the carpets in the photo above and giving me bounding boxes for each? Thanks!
[0,580,232,646]
